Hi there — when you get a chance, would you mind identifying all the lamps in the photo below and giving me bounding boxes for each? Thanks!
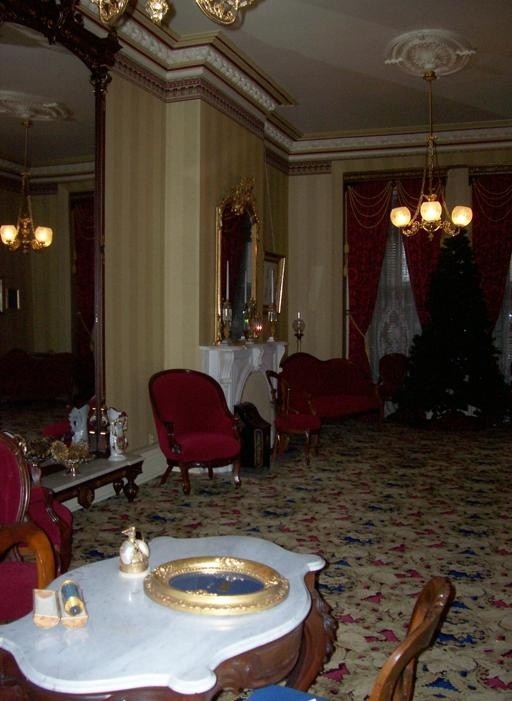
[0,107,54,254]
[388,72,473,240]
[95,0,251,28]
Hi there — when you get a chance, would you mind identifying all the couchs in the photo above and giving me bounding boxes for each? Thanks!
[0,348,92,414]
[278,352,383,446]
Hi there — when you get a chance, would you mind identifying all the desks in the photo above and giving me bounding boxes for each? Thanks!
[40,451,143,508]
[0,533,338,701]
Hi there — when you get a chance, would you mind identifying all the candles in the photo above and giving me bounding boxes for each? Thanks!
[244,270,247,304]
[271,268,275,305]
[226,259,230,301]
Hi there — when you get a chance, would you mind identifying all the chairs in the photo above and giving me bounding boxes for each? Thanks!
[376,353,412,418]
[0,428,73,626]
[148,369,243,494]
[1,521,56,701]
[241,579,450,701]
[265,370,321,466]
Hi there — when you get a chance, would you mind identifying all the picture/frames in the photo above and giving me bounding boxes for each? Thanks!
[264,250,285,315]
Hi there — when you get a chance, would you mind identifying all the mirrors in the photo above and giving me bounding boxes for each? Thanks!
[217,175,264,344]
[0,71,106,478]
